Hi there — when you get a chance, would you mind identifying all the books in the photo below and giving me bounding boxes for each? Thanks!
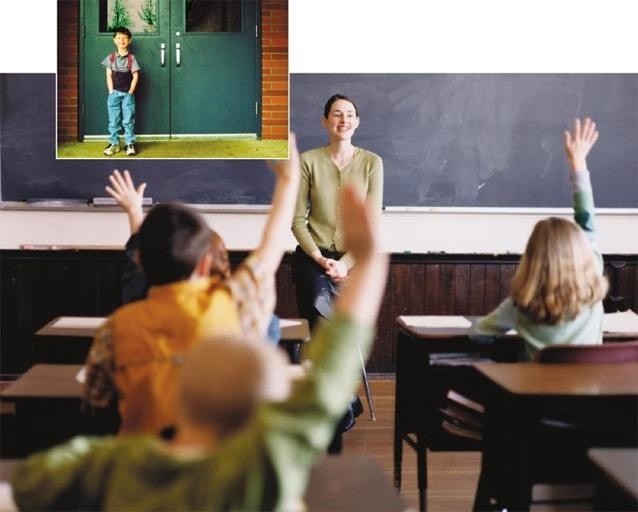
[602,308,638,339]
[427,351,494,441]
[529,501,592,512]
[529,481,595,501]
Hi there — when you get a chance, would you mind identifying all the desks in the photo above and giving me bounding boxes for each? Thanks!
[0,364,84,416]
[393,315,638,512]
[0,315,310,363]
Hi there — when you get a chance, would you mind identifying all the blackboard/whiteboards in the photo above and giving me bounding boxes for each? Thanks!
[0,73,638,214]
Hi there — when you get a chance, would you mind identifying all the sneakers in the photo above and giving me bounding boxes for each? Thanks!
[104,145,120,156]
[124,145,135,155]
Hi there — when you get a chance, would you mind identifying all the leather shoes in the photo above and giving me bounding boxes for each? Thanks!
[327,409,355,454]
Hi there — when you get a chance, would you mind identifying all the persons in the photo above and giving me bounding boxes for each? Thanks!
[102,167,281,348]
[12,177,392,512]
[79,129,301,446]
[99,27,140,156]
[464,118,609,431]
[291,93,384,456]
